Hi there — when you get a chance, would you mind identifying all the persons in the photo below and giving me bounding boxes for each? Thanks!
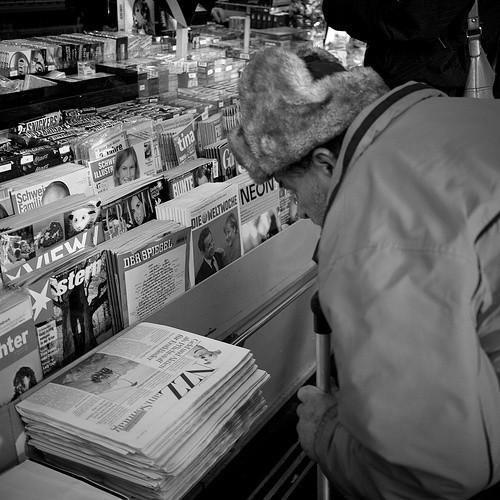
[226,41,500,500]
[319,2,476,94]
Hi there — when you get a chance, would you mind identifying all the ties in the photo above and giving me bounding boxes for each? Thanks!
[210,258,217,271]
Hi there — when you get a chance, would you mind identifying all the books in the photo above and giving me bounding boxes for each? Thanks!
[1,0,351,499]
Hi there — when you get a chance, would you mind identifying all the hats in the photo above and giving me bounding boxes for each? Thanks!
[226,43,392,185]
